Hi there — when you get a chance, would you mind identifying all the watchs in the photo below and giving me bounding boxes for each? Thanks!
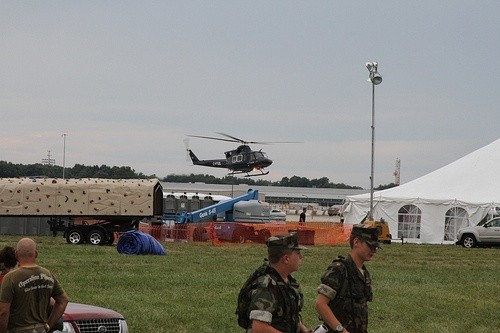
[334,325,344,333]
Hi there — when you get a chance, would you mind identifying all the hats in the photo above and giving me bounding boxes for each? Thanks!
[265,232,309,250]
[351,223,381,248]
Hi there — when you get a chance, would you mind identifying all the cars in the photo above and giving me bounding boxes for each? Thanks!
[455,216,500,248]
[61,302,130,333]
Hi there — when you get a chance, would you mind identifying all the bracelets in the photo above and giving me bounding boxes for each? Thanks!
[46,323,50,332]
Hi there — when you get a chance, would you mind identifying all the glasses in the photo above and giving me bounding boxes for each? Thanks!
[365,241,375,248]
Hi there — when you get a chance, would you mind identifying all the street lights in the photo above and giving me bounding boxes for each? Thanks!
[365,60,383,221]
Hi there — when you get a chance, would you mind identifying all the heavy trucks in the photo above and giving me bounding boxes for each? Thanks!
[0,178,164,246]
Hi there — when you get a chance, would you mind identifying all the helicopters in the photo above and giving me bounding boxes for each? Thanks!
[181,132,304,177]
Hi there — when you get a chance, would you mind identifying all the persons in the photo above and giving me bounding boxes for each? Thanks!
[247,232,314,333]
[299,207,307,225]
[0,238,68,333]
[314,224,384,333]
[0,246,18,285]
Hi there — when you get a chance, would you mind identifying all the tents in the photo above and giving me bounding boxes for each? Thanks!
[343,138,500,245]
